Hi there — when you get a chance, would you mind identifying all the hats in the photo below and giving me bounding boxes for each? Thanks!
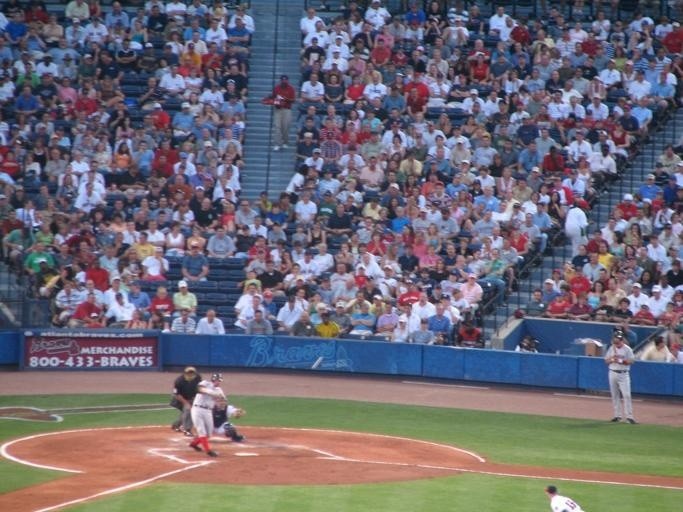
[631,281,662,309]
[613,326,625,341]
[184,366,223,382]
[544,484,556,494]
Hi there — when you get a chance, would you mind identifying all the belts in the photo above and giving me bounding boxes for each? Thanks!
[192,405,214,412]
[609,369,629,373]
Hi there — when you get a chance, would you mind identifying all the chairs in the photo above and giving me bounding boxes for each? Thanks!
[0,1,683,347]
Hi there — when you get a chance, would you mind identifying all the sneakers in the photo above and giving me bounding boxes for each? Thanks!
[611,417,638,425]
[170,423,247,458]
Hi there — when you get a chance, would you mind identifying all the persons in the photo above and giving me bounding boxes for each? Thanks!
[169,365,202,436]
[545,484,584,512]
[604,330,639,425]
[212,393,246,441]
[186,372,228,458]
